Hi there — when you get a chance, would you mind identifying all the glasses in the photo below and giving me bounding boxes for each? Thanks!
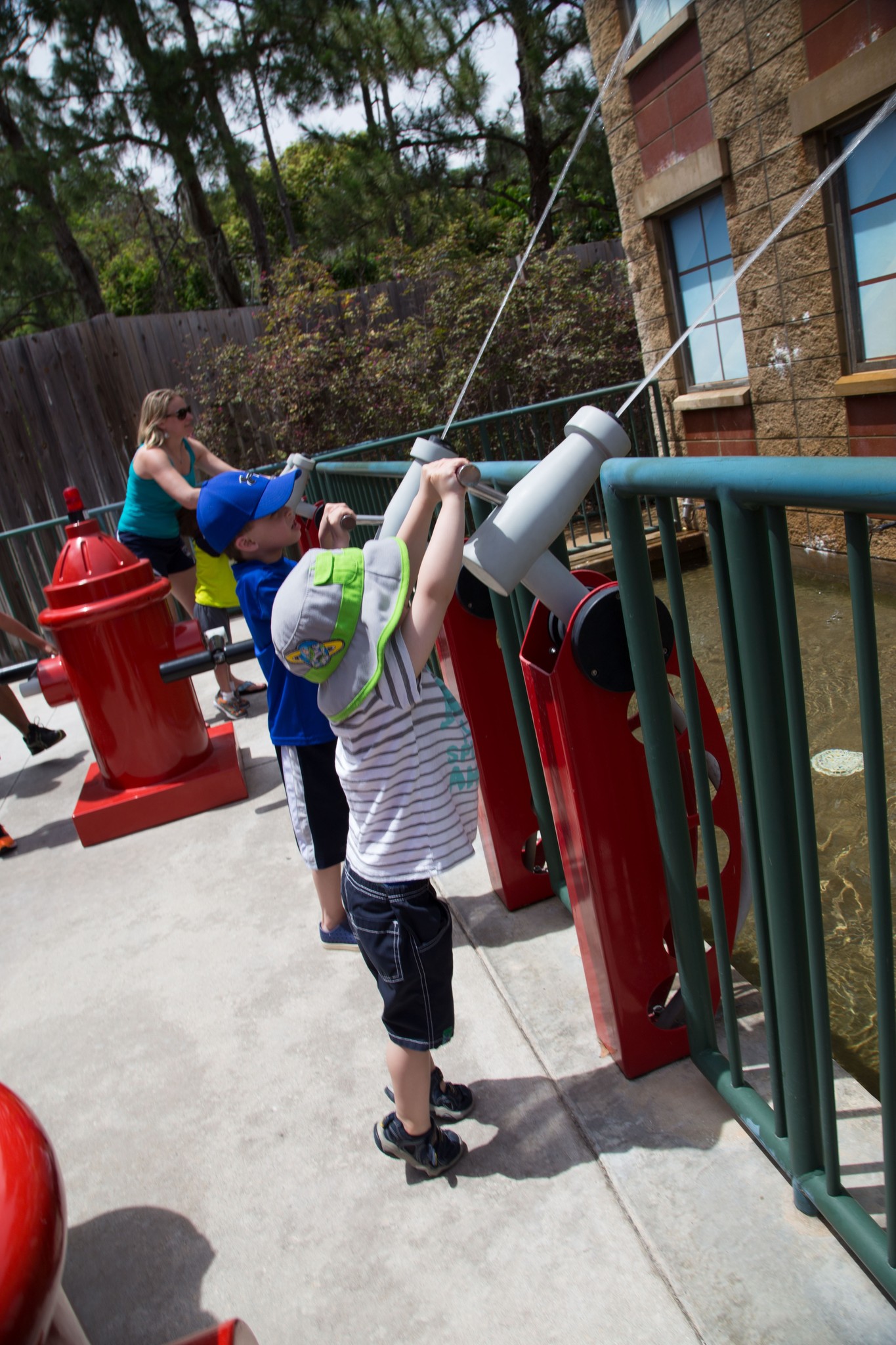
[164,404,193,421]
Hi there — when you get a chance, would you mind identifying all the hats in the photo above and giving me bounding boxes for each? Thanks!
[269,534,411,728]
[197,468,302,556]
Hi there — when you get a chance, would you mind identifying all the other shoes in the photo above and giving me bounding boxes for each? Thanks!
[319,917,361,950]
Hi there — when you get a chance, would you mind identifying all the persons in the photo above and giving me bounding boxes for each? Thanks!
[116,390,266,720]
[197,469,368,947]
[269,457,480,1178]
[0,614,66,856]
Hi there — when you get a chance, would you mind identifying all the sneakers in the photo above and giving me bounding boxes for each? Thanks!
[21,715,66,756]
[0,824,17,857]
[373,1112,464,1178]
[212,692,250,719]
[384,1066,477,1121]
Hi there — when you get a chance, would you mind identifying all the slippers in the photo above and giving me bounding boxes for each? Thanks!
[235,680,267,696]
